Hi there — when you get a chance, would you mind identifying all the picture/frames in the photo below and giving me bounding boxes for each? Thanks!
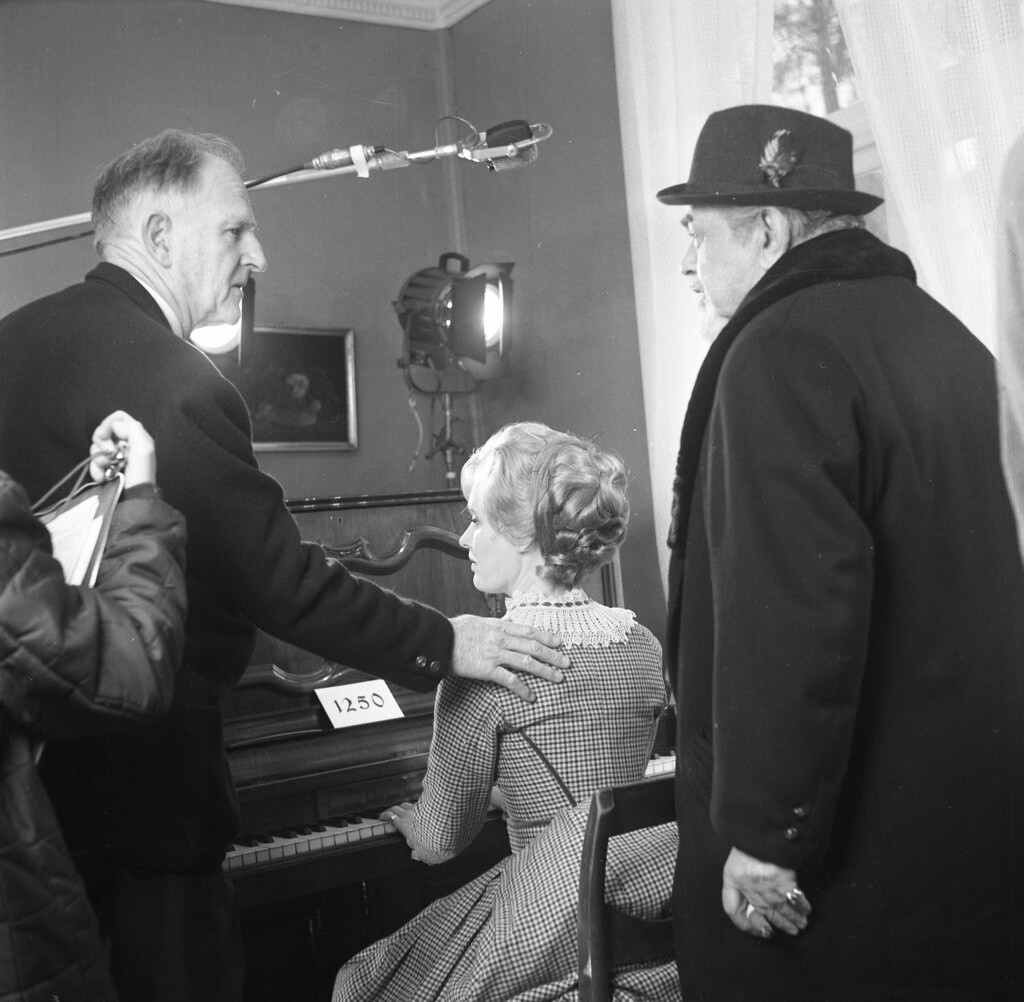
[232,322,360,453]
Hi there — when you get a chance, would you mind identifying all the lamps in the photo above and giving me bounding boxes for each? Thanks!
[392,252,515,492]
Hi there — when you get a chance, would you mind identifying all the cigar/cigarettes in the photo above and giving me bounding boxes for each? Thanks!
[745,903,773,938]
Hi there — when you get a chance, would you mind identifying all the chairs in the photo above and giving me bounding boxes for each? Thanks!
[576,775,678,1002]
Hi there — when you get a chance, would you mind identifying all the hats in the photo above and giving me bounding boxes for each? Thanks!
[655,105,885,216]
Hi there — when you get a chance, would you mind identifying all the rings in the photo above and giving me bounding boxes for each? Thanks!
[389,814,397,824]
[785,889,802,906]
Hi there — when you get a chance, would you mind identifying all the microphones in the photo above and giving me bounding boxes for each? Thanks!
[484,119,538,172]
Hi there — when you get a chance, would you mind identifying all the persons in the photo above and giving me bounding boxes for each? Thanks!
[0,410,189,1002]
[330,421,683,1002]
[658,104,1024,1002]
[0,125,574,1002]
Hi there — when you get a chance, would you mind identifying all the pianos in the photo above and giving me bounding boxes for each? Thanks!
[212,491,683,1002]
